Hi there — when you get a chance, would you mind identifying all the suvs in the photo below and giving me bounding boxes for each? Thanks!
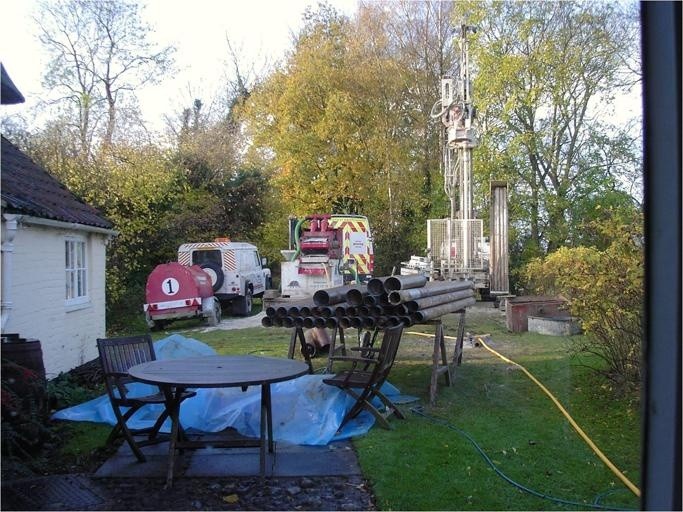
[175,238,274,318]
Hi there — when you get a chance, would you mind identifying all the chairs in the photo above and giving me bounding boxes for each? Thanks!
[322,321,406,430]
[96,335,197,463]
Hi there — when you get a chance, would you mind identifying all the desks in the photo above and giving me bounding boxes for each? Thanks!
[128,354,310,488]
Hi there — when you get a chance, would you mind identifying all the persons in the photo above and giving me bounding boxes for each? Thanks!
[262,265,273,289]
